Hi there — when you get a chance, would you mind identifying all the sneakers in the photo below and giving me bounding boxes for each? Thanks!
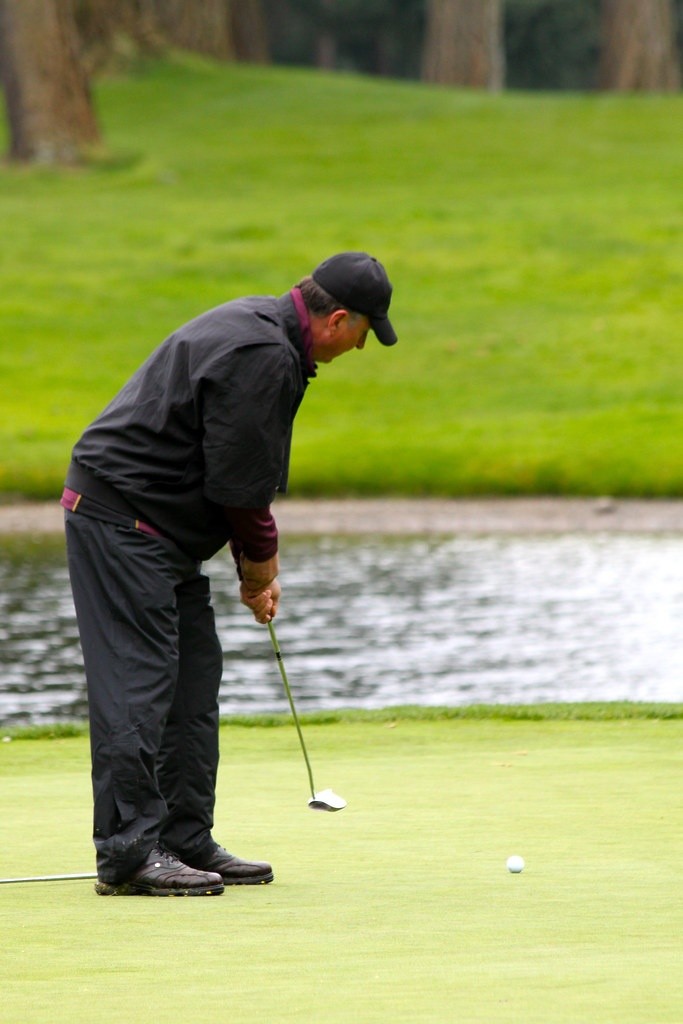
[185,842,275,885]
[94,848,226,896]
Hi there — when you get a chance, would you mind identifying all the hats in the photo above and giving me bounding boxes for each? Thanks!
[315,252,397,346]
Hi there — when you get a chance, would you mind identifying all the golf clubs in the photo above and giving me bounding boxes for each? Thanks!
[265,615,346,815]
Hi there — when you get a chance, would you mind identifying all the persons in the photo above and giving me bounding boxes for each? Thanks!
[58,250,399,900]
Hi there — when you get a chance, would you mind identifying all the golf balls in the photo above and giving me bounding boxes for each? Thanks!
[505,854,524,877]
